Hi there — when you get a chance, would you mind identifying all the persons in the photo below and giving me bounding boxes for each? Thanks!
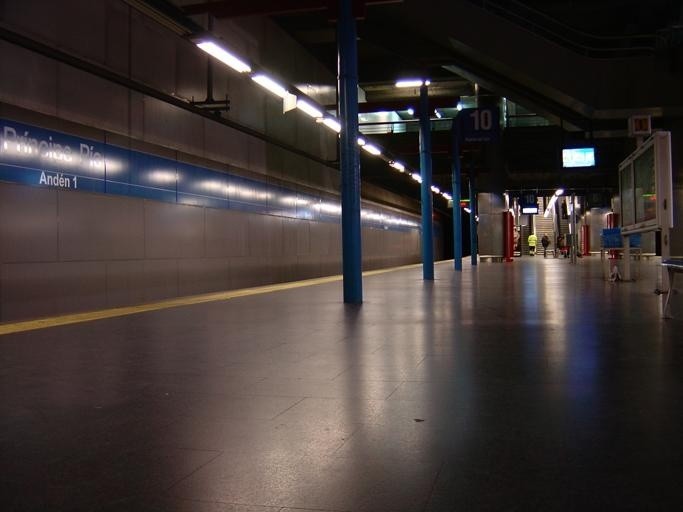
[541,233,551,257]
[560,200,567,219]
[556,232,563,248]
[527,232,537,256]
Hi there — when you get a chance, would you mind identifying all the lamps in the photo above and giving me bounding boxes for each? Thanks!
[434,108,446,119]
[185,29,384,158]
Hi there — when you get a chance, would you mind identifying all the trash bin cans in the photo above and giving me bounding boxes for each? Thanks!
[610,248,641,282]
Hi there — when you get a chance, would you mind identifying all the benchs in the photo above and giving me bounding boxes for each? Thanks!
[588,251,608,257]
[619,253,656,260]
[480,254,503,262]
